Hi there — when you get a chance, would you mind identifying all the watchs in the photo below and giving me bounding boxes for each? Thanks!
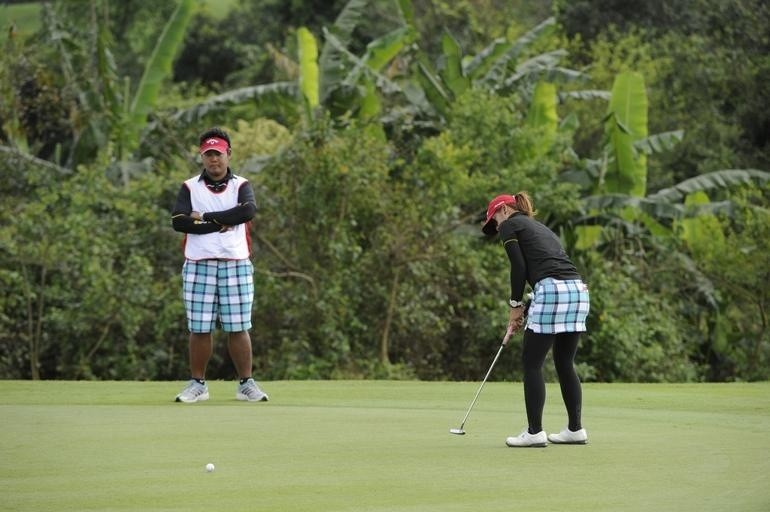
[509,300,522,307]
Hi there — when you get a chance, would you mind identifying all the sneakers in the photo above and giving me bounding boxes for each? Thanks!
[506,430,548,447]
[175,379,209,403]
[548,428,587,444]
[237,378,268,402]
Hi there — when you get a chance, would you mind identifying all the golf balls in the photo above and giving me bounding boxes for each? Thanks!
[206,463,214,472]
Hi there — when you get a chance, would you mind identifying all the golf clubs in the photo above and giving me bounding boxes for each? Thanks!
[449,325,512,434]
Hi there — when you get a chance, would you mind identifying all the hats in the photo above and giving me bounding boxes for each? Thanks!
[200,136,228,156]
[482,195,517,235]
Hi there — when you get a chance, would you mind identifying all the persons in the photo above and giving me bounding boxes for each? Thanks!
[482,193,588,446]
[171,129,269,403]
